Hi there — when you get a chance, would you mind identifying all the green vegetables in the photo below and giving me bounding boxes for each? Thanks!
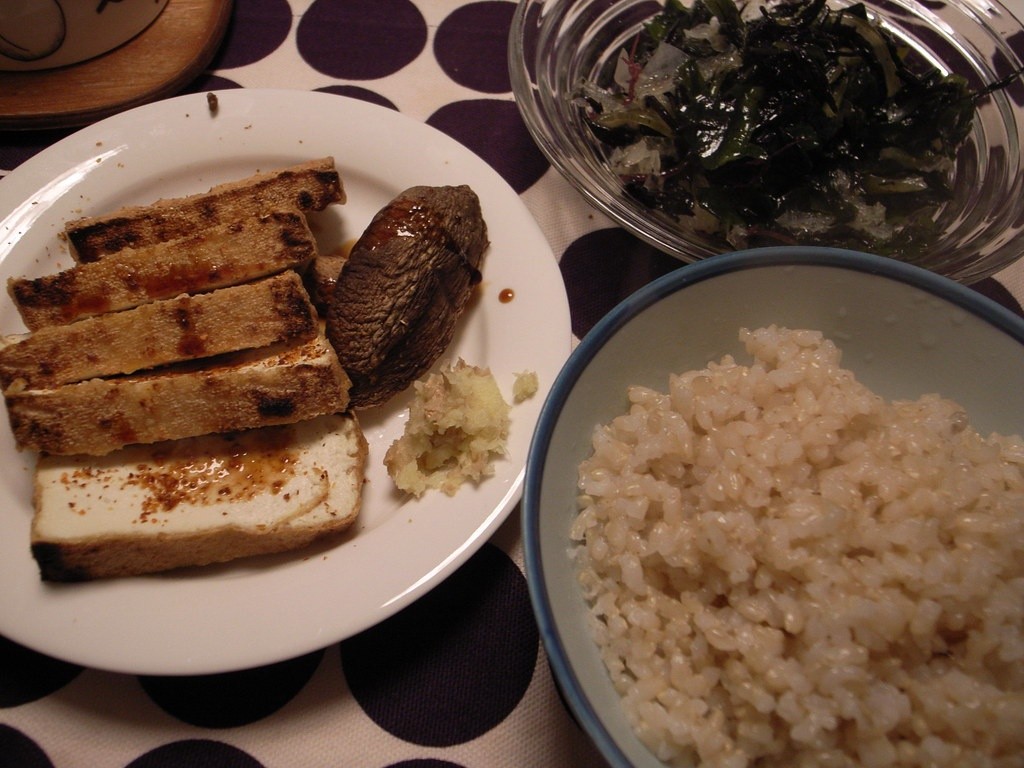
[583,0,1024,248]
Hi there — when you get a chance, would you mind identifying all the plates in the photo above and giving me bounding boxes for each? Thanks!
[0,87,575,676]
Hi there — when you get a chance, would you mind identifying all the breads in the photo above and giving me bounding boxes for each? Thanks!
[0,156,371,582]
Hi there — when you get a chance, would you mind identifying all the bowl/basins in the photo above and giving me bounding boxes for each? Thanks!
[518,244,1024,768]
[505,0,1024,288]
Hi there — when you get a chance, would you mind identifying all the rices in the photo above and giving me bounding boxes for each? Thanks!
[572,325,1024,768]
